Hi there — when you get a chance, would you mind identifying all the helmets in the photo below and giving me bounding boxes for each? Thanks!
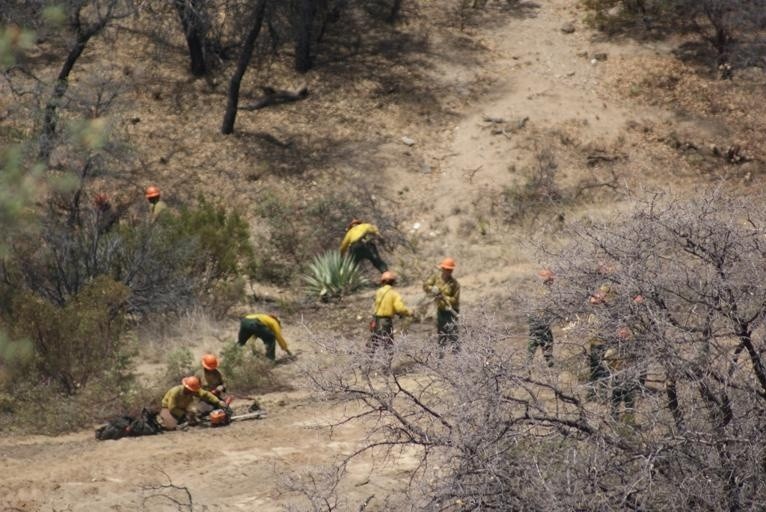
[182,376,200,394]
[202,354,220,372]
[381,272,397,281]
[347,218,359,227]
[144,186,161,199]
[439,257,457,270]
[538,269,558,283]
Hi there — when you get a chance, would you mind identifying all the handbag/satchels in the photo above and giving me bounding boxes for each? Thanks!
[96,409,158,440]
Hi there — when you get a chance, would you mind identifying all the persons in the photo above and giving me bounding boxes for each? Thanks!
[360,272,415,382]
[194,354,229,421]
[238,313,297,368]
[145,186,168,217]
[155,375,233,431]
[423,258,462,368]
[521,268,558,381]
[338,216,395,275]
[95,191,120,232]
[585,257,651,440]
